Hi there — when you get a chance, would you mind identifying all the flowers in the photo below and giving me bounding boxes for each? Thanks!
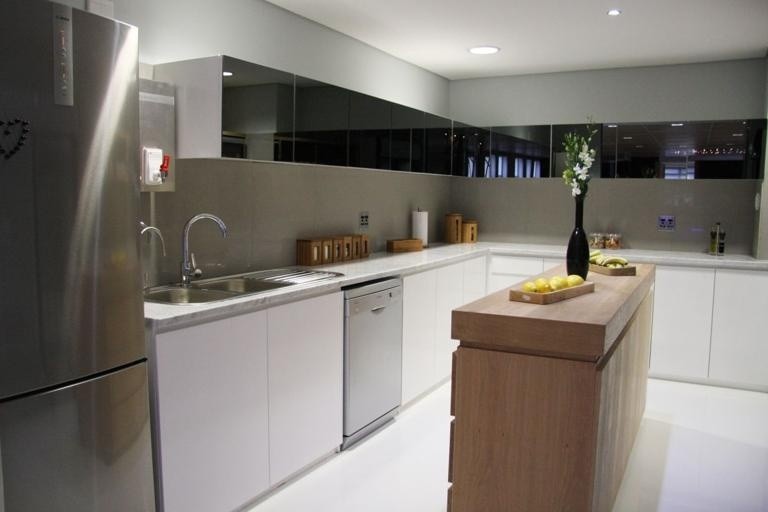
[560,113,600,198]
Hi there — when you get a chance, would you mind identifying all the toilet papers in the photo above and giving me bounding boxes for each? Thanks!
[411,210,428,246]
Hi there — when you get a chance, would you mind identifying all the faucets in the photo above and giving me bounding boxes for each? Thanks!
[140,221,166,257]
[181,213,230,285]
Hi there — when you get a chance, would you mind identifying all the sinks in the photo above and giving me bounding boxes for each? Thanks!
[193,276,297,295]
[142,284,243,305]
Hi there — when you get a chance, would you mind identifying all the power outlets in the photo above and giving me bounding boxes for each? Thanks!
[358,212,370,229]
[655,214,676,232]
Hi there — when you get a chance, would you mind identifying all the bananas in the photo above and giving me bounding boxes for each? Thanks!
[589,250,627,269]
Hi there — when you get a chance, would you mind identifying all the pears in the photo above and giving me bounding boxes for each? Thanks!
[523,275,584,293]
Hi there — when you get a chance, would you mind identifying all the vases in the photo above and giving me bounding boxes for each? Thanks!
[565,194,589,285]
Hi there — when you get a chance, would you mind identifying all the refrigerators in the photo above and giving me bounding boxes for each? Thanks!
[0,0,158,510]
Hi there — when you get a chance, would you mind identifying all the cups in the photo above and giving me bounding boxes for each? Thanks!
[587,232,624,250]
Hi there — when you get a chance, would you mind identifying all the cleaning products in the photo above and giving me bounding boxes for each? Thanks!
[708,221,726,257]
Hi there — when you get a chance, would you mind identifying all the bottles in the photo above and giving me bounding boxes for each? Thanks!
[708,226,726,256]
[445,211,462,242]
[463,220,479,242]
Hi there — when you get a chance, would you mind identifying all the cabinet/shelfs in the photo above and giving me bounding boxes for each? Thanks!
[646,261,764,397]
[490,254,560,296]
[395,249,489,408]
[442,258,660,509]
[602,117,766,179]
[449,118,600,183]
[146,292,342,512]
[158,54,349,174]
[348,90,452,176]
